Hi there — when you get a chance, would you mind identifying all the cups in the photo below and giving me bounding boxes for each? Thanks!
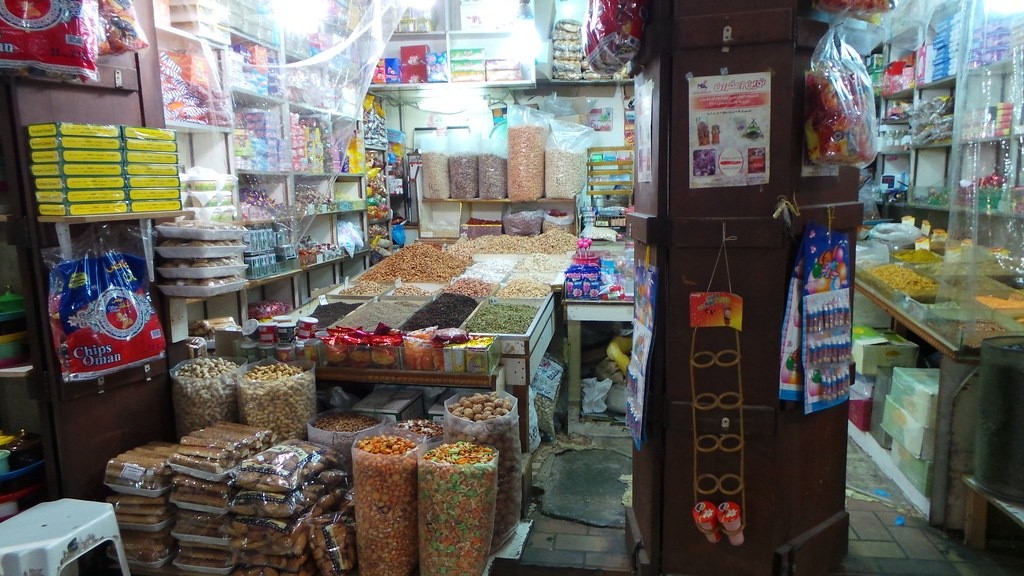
[241,315,319,362]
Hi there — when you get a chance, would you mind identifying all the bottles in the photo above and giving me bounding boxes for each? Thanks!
[299,236,336,265]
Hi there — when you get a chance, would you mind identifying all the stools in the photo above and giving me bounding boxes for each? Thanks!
[1,497,132,576]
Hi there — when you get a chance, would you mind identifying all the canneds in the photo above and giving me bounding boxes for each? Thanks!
[239,315,321,367]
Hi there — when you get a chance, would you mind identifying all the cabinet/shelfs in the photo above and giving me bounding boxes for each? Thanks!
[153,0,535,343]
[853,0,1024,220]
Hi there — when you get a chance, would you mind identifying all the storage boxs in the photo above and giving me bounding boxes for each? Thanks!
[278,259,556,386]
[420,200,471,238]
[850,326,939,497]
[427,388,491,422]
[460,222,502,238]
[353,386,424,424]
[856,225,1023,357]
[866,54,884,87]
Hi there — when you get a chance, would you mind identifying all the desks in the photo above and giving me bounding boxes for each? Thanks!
[563,299,633,438]
[854,278,980,528]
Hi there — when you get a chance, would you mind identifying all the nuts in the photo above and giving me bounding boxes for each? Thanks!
[443,390,522,557]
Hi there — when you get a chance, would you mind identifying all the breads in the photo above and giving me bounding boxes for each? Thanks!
[105,421,358,576]
